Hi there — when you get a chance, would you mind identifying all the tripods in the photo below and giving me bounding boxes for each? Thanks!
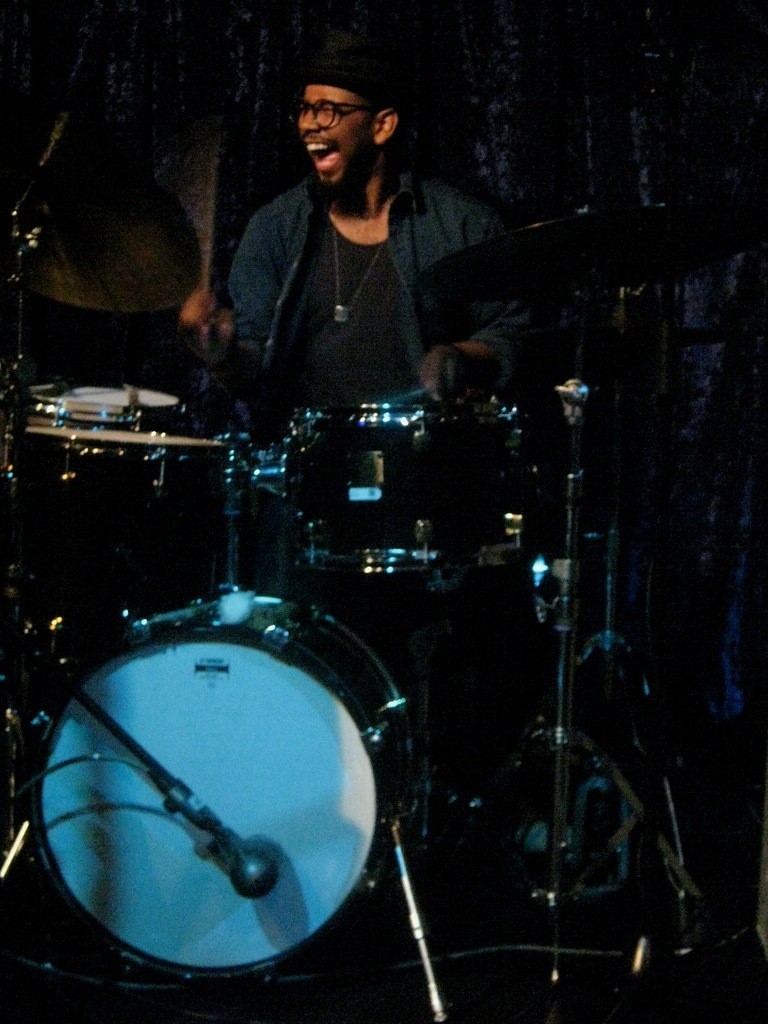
[425,378,737,993]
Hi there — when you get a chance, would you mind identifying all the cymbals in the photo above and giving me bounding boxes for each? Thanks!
[418,194,701,302]
[0,165,199,314]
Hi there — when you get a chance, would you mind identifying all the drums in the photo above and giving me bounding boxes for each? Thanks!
[31,382,182,494]
[32,589,417,980]
[290,385,530,572]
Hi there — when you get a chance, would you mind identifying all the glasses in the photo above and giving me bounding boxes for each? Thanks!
[287,98,382,129]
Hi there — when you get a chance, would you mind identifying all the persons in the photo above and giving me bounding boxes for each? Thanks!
[179,26,548,413]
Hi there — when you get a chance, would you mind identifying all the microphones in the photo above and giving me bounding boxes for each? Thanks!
[143,761,280,899]
[37,109,73,169]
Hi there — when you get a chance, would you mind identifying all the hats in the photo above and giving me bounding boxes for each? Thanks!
[296,29,403,105]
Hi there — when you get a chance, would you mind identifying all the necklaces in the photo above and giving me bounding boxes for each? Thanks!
[332,205,386,323]
